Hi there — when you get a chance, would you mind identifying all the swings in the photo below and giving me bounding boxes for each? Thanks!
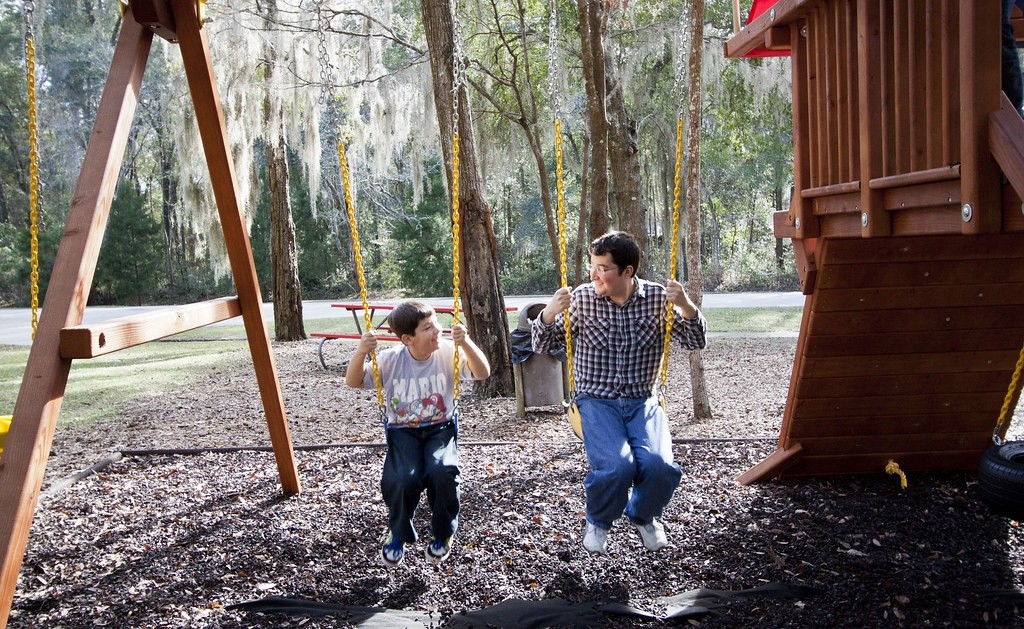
[976,345,1024,523]
[315,0,462,453]
[547,0,690,440]
[0,0,48,451]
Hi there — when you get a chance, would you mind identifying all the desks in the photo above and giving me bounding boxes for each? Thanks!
[331,303,518,361]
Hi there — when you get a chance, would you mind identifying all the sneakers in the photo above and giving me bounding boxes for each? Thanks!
[425,513,458,563]
[622,503,669,552]
[581,501,610,556]
[381,523,407,568]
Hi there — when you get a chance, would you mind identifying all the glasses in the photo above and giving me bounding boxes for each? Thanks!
[586,263,618,275]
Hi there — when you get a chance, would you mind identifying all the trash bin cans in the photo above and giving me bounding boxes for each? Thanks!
[517,301,565,408]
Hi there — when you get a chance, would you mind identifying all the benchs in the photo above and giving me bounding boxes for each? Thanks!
[376,326,451,334]
[310,333,402,370]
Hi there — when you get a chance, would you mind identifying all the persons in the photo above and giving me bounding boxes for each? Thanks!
[346,301,490,567]
[532,231,707,554]
